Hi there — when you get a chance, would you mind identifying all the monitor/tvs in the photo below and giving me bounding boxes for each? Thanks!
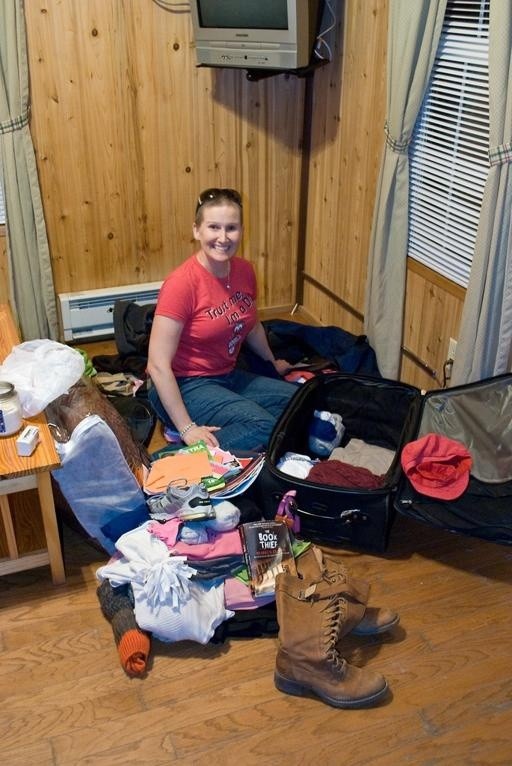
[188,0,308,70]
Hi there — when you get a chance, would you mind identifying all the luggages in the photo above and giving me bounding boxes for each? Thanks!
[261,374,511,554]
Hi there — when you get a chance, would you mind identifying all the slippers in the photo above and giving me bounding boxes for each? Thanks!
[111,397,153,446]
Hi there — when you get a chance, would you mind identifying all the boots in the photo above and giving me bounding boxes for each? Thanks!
[275,572,387,706]
[294,547,399,635]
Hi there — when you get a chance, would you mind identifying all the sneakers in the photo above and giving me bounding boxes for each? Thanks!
[149,484,215,522]
[147,417,170,455]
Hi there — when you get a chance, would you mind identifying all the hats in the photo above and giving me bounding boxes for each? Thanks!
[402,434,473,500]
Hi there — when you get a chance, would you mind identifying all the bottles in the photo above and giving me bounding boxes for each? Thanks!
[0,381,25,439]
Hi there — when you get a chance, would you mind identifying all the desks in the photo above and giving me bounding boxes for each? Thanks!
[0,302,67,587]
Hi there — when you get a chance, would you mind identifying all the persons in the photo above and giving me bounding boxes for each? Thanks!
[145,188,302,451]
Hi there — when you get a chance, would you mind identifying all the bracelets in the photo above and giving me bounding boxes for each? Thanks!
[180,422,196,440]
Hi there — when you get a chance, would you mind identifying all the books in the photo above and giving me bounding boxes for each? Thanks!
[143,437,268,500]
[237,517,300,599]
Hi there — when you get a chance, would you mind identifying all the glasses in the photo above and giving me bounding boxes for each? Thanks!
[196,189,242,216]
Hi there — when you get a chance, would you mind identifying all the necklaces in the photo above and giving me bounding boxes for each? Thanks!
[198,250,232,291]
